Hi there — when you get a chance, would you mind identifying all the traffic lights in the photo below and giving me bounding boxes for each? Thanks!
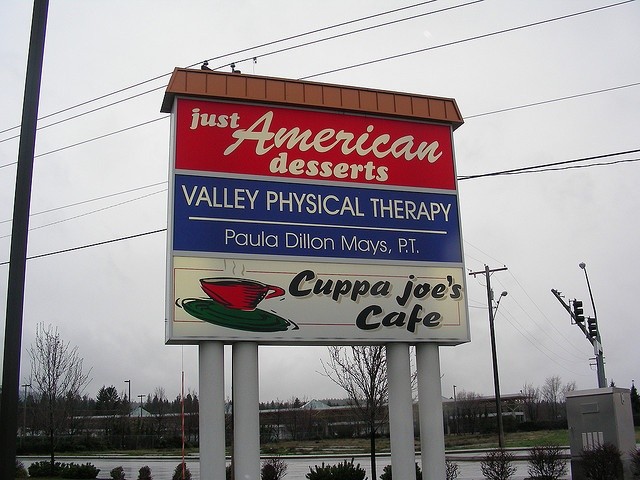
[587,317,600,336]
[572,300,586,327]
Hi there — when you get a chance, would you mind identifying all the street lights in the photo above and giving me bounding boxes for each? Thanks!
[491,290,509,445]
[579,263,608,389]
